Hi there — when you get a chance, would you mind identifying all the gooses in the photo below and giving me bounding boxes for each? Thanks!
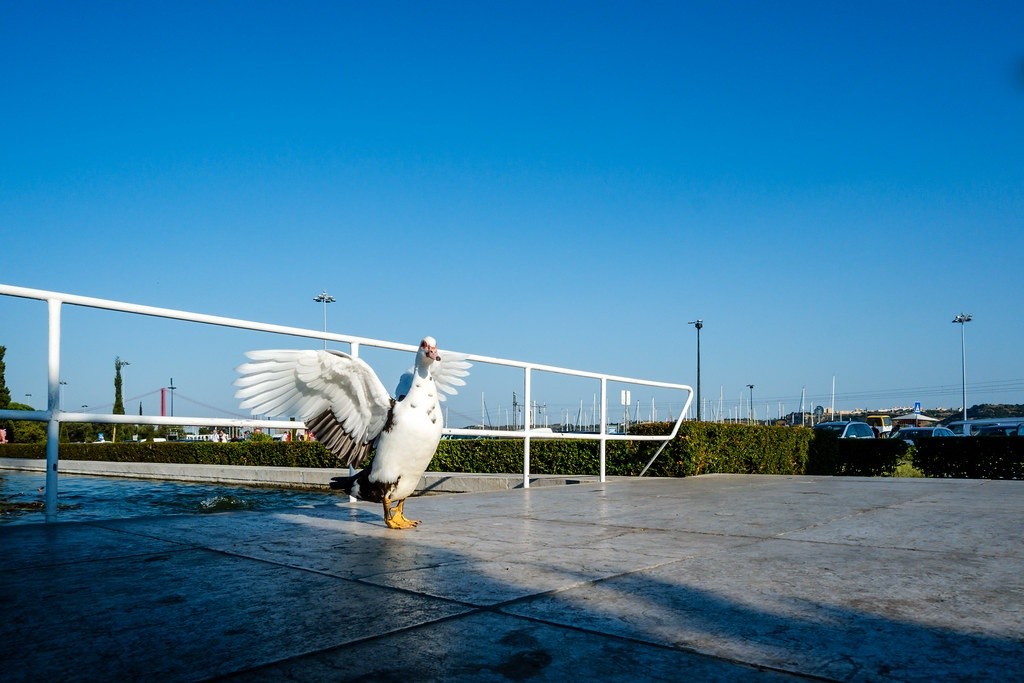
[233,336,473,530]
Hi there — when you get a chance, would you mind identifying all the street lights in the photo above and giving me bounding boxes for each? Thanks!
[25,394,32,405]
[687,319,703,420]
[746,384,756,425]
[313,291,336,350]
[952,313,972,420]
[59,380,67,412]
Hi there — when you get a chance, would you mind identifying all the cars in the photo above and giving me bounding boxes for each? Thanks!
[945,416,1024,436]
[813,420,875,438]
[890,426,957,439]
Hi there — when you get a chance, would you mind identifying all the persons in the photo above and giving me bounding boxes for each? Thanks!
[303,430,315,441]
[282,431,291,442]
[244,431,252,441]
[211,429,228,444]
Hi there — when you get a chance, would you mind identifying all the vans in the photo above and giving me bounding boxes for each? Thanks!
[866,415,893,438]
